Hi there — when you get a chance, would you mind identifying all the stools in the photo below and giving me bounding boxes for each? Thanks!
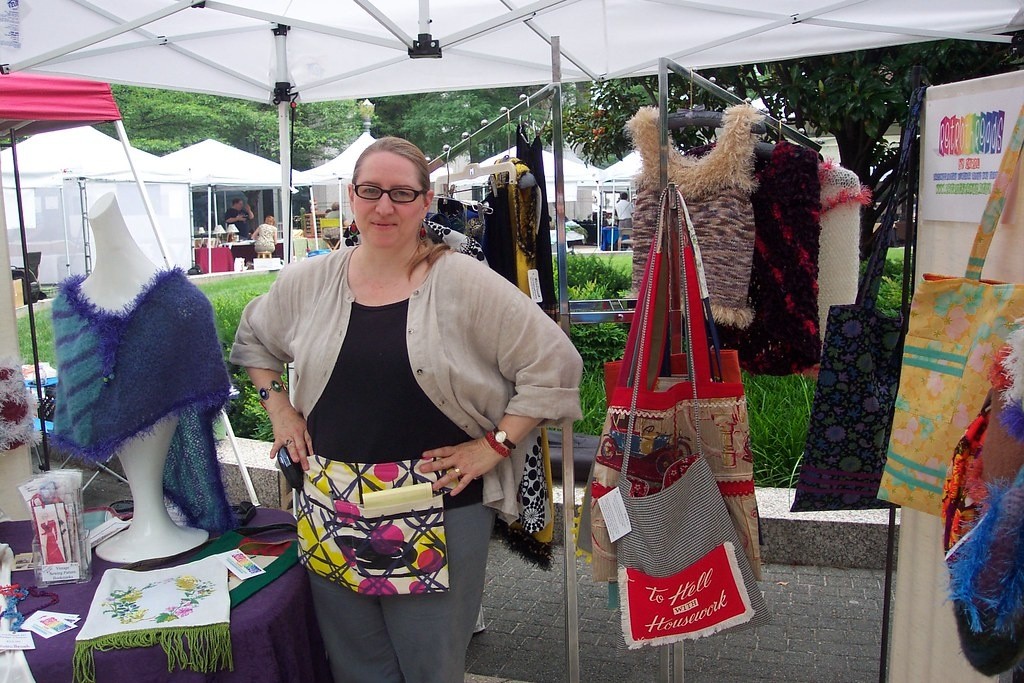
[257,251,273,258]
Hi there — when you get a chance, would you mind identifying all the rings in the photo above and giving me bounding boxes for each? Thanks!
[284,439,294,447]
[453,468,461,475]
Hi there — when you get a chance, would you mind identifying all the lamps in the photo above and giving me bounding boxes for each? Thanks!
[195,226,206,248]
[212,225,226,248]
[226,224,240,243]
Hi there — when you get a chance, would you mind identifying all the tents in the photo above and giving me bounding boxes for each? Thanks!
[0,0,1024,505]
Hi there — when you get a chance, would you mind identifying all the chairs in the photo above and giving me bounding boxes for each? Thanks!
[320,218,348,251]
[617,228,633,251]
[23,378,129,493]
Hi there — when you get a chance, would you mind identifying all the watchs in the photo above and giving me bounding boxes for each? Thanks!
[493,427,516,449]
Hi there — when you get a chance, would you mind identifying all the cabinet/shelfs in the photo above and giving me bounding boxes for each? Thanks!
[304,212,326,238]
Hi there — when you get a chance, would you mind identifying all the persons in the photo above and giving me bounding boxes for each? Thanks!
[614,192,633,250]
[229,136,585,683]
[225,198,254,260]
[324,202,348,247]
[350,223,358,236]
[50,191,237,567]
[606,213,612,227]
[250,216,277,253]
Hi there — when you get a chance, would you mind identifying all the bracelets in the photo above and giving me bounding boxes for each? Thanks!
[485,430,513,458]
[259,380,287,410]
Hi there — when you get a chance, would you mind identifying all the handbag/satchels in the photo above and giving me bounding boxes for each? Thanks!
[574,186,771,649]
[877,104,1024,517]
[789,85,927,513]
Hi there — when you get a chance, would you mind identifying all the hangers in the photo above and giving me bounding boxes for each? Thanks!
[500,107,514,163]
[621,68,767,140]
[433,147,493,225]
[435,133,517,196]
[519,95,541,139]
[743,99,776,159]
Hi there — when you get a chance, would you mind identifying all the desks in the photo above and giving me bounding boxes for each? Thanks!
[0,508,331,683]
[222,243,284,266]
[195,247,234,273]
[600,226,629,251]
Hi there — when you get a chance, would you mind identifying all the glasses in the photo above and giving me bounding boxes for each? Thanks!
[352,184,424,203]
